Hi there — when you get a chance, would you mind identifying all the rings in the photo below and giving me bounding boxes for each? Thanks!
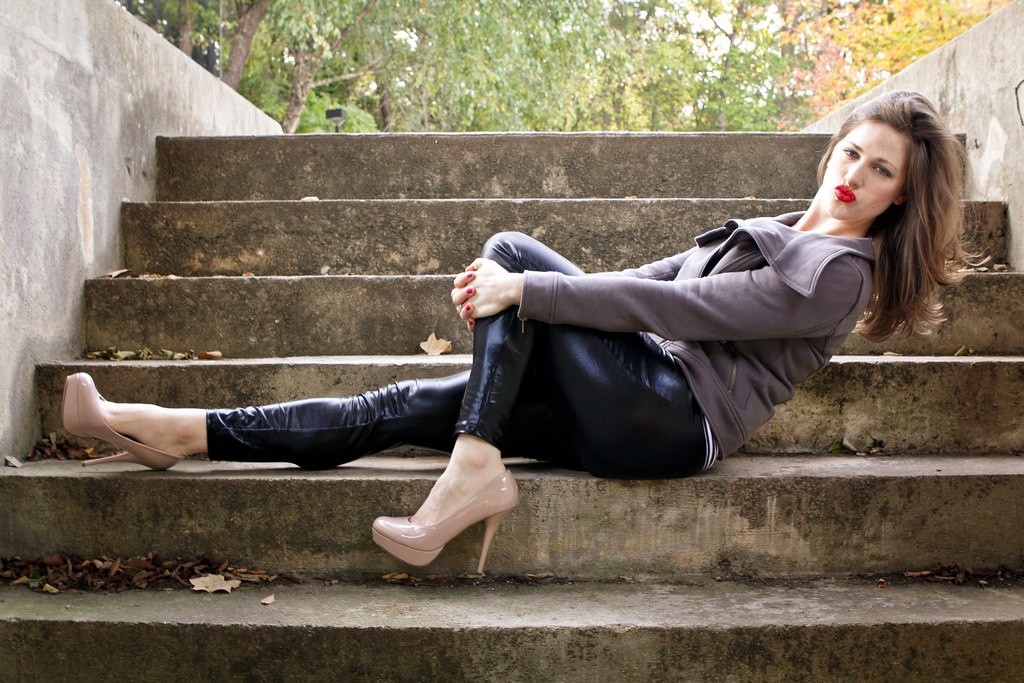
[459,304,464,308]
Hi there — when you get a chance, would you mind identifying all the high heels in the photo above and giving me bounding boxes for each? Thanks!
[372,469,519,573]
[62,373,186,470]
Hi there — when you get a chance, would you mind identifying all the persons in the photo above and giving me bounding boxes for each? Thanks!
[62,92,977,574]
